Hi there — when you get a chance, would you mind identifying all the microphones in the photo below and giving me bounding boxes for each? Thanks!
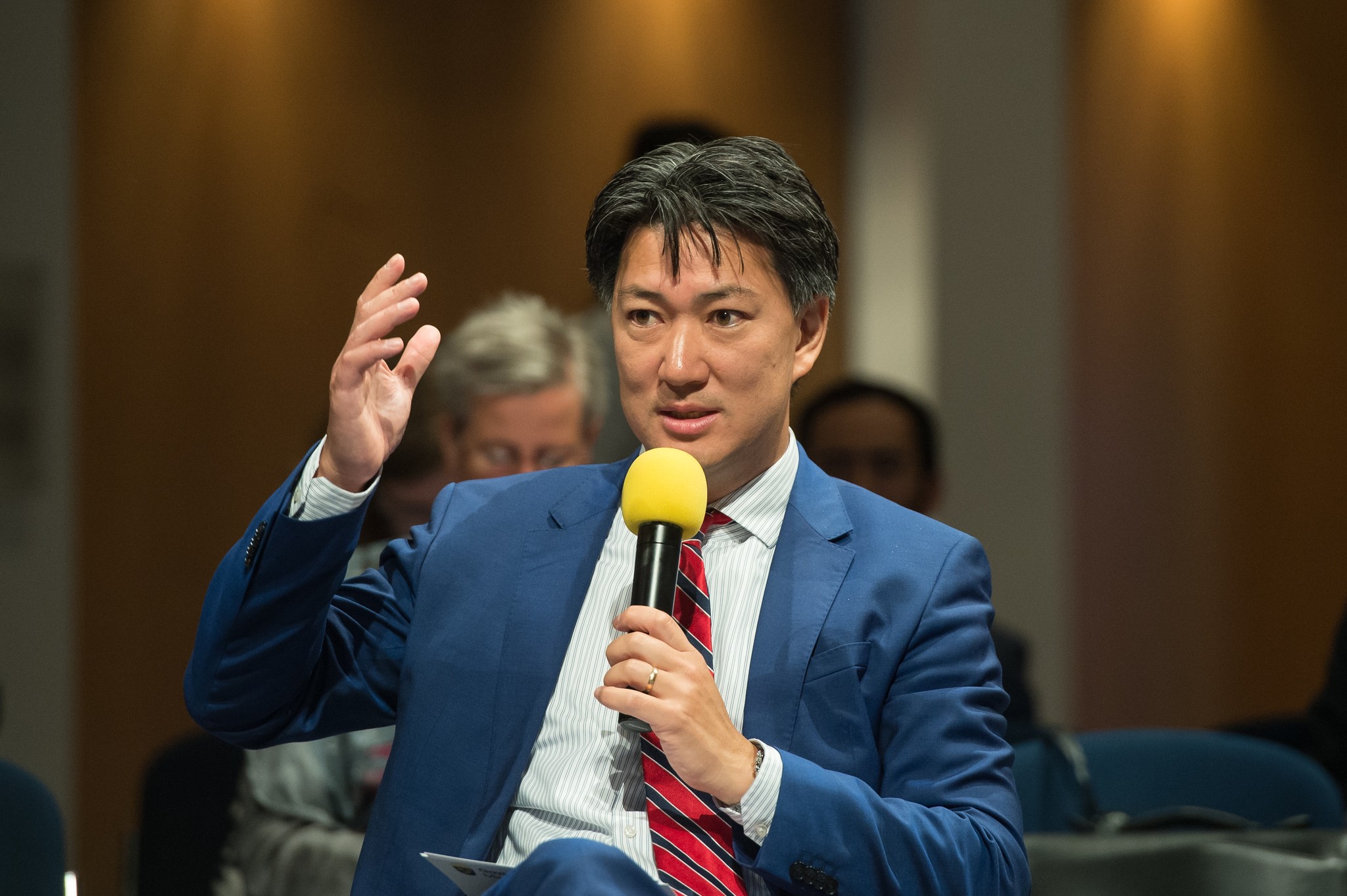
[619,447,709,729]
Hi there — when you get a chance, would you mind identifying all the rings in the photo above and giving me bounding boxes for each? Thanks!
[642,666,658,695]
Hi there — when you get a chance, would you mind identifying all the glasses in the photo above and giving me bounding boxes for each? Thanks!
[457,444,591,480]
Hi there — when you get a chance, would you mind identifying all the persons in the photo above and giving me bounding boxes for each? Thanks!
[182,136,1034,896]
[796,379,1104,836]
[222,293,609,896]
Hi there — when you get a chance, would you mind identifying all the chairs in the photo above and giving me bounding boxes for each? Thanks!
[1009,727,1347,833]
[131,733,246,896]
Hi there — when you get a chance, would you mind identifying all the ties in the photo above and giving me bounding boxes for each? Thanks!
[639,507,745,896]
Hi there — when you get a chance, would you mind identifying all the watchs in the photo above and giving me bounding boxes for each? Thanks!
[722,745,764,814]
